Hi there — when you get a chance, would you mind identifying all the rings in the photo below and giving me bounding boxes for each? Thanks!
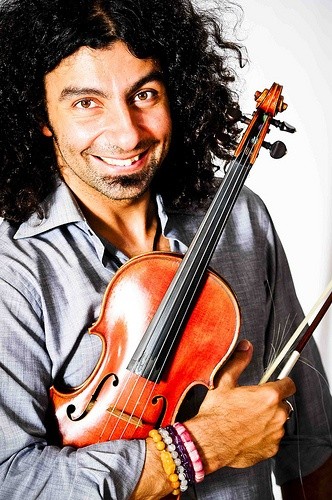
[284,399,294,420]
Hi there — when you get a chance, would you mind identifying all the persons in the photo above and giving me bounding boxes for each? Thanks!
[1,0,332,500]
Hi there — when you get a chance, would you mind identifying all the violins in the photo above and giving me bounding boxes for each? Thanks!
[44,81,296,448]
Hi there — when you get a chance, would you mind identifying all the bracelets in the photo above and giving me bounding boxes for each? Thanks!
[150,421,206,496]
